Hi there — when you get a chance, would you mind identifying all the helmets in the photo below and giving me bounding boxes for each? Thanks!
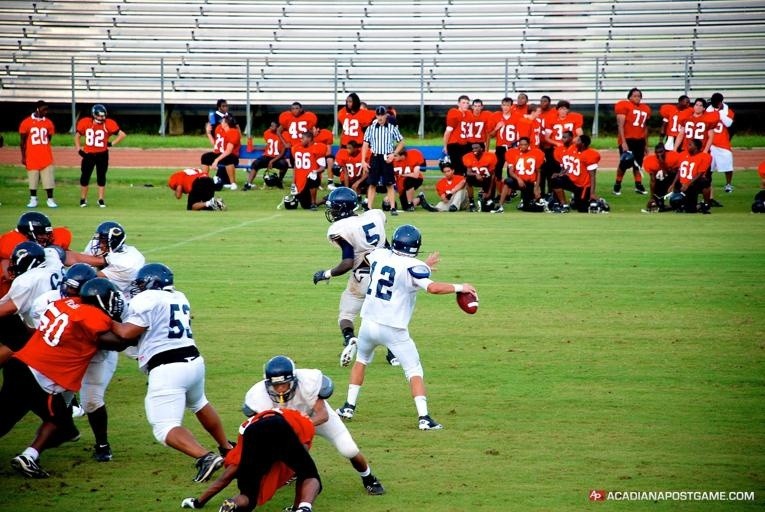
[263,355,298,404]
[391,224,422,257]
[18,211,175,319]
[92,104,108,122]
[284,196,298,209]
[382,195,397,211]
[263,172,280,187]
[324,186,358,224]
[438,158,452,173]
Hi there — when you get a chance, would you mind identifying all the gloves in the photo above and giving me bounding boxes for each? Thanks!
[180,496,201,509]
[313,271,329,285]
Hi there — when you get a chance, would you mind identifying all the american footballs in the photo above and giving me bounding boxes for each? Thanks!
[456,291,477,314]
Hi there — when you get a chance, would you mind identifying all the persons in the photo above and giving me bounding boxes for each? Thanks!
[18,100,59,209]
[334,224,477,431]
[181,410,325,512]
[168,87,765,217]
[241,355,385,496]
[312,187,401,369]
[112,261,237,483]
[0,211,146,480]
[73,103,127,208]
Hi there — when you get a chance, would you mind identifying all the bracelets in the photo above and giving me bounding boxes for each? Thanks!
[452,283,464,294]
[107,141,112,147]
[79,150,86,157]
[323,269,332,279]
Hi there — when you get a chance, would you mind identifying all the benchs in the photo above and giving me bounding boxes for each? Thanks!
[217,144,444,191]
[0,0,765,134]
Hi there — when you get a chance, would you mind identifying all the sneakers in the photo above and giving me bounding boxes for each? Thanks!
[218,441,237,457]
[231,182,238,190]
[80,198,87,209]
[295,504,313,512]
[360,473,385,495]
[634,184,649,195]
[241,181,252,191]
[611,187,621,194]
[27,195,39,208]
[209,197,227,211]
[466,192,610,214]
[11,453,51,476]
[47,197,59,208]
[339,336,359,368]
[386,350,400,366]
[418,415,444,430]
[642,197,722,215]
[66,425,81,443]
[97,199,105,208]
[72,402,86,418]
[192,451,225,484]
[336,402,356,420]
[389,207,399,216]
[94,442,114,462]
[219,498,237,512]
[725,184,733,193]
